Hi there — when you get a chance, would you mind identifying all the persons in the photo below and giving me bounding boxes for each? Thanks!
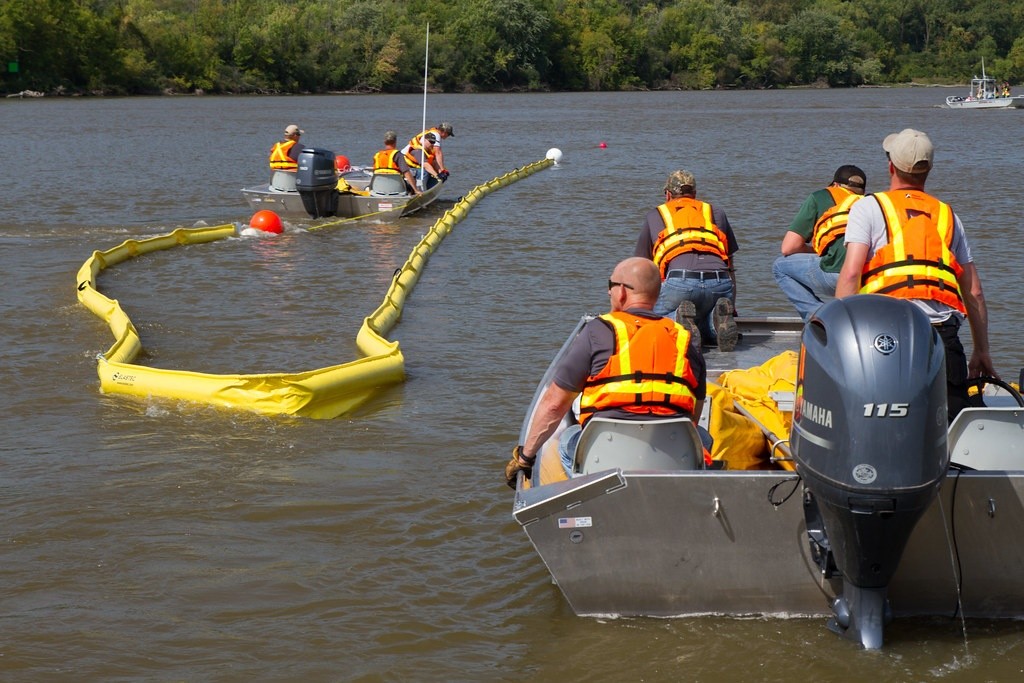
[400,122,456,188]
[835,128,1000,426]
[269,124,305,192]
[1001,81,1011,97]
[633,169,739,352]
[373,130,423,197]
[773,165,867,325]
[505,257,723,491]
[995,87,999,98]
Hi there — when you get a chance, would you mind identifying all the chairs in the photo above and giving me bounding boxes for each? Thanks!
[944,407,1024,470]
[269,170,298,192]
[369,173,407,195]
[572,416,707,478]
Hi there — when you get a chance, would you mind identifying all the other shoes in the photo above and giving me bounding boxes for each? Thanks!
[676,300,701,354]
[713,297,738,352]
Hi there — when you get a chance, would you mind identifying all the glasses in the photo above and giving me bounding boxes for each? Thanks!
[444,131,451,136]
[429,140,436,144]
[609,279,635,292]
[296,133,300,136]
[885,151,892,161]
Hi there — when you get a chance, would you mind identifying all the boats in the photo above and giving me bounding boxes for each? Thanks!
[945,55,1024,110]
[510,285,1024,652]
[240,19,447,224]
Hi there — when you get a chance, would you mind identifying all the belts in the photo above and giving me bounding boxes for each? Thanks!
[668,271,730,280]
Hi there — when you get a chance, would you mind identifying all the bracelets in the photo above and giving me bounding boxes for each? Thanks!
[518,447,536,461]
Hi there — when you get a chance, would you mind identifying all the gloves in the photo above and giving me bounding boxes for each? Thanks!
[504,446,532,491]
[441,169,449,176]
[436,176,443,183]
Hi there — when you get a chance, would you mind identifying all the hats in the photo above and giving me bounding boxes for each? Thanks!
[285,124,304,136]
[665,170,697,197]
[439,123,455,137]
[384,131,397,142]
[883,128,934,173]
[424,133,439,142]
[829,165,866,191]
